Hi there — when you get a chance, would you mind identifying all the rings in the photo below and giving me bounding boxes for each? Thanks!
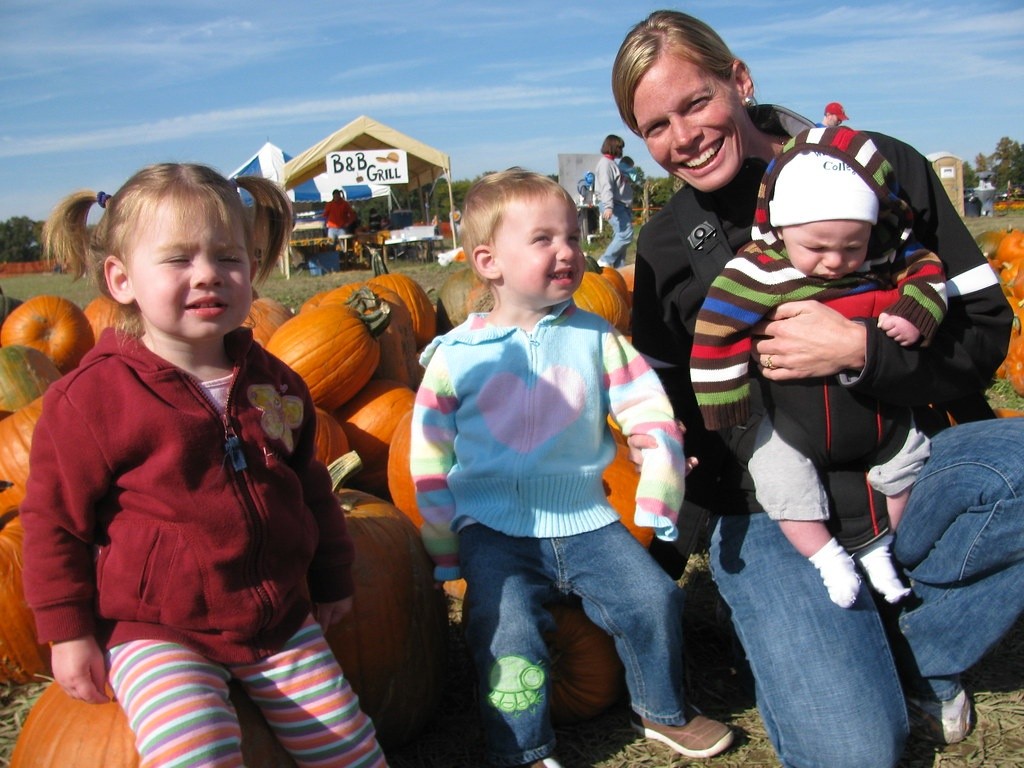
[766,356,777,369]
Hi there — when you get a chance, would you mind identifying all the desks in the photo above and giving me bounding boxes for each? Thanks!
[380,235,443,265]
[279,238,333,279]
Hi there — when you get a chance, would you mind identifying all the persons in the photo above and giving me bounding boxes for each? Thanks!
[18,165,391,767]
[594,135,634,268]
[432,215,442,235]
[404,164,733,768]
[448,204,461,235]
[815,103,850,128]
[368,208,383,231]
[322,190,355,253]
[613,11,1023,768]
[689,126,948,610]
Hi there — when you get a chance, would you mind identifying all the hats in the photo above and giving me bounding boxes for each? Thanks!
[769,151,879,228]
[825,103,849,121]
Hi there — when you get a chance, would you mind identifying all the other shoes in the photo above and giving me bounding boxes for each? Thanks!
[597,258,610,267]
[630,705,734,758]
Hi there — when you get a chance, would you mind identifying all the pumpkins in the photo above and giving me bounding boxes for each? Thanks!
[971,225,1024,419]
[0,290,289,768]
[237,253,658,746]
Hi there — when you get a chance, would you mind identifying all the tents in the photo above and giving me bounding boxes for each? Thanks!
[279,115,458,281]
[225,142,393,214]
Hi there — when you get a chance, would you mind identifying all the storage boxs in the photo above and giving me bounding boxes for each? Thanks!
[390,226,434,239]
[308,251,339,276]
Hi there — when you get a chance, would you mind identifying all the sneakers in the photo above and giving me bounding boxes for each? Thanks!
[904,687,972,744]
